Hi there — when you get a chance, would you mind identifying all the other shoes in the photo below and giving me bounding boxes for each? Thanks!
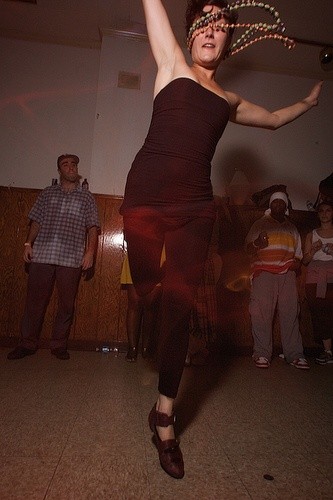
[314,352,333,367]
[51,348,69,360]
[7,347,36,359]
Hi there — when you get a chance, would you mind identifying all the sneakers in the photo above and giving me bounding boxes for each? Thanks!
[254,356,271,367]
[290,358,310,369]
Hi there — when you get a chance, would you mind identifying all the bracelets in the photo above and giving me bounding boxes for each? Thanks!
[252,239,259,249]
[22,241,31,247]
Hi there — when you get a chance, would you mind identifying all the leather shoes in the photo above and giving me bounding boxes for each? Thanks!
[148,402,184,479]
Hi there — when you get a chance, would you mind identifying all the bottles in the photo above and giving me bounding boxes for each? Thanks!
[82,178,89,191]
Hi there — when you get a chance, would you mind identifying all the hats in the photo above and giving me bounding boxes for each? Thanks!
[269,191,288,209]
[57,154,80,166]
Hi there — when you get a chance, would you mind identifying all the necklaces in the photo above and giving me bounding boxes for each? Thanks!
[187,0,296,67]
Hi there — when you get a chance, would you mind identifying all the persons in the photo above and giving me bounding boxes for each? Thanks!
[176,241,222,366]
[303,201,333,367]
[5,154,99,360]
[243,191,309,370]
[122,0,324,480]
[118,240,167,364]
